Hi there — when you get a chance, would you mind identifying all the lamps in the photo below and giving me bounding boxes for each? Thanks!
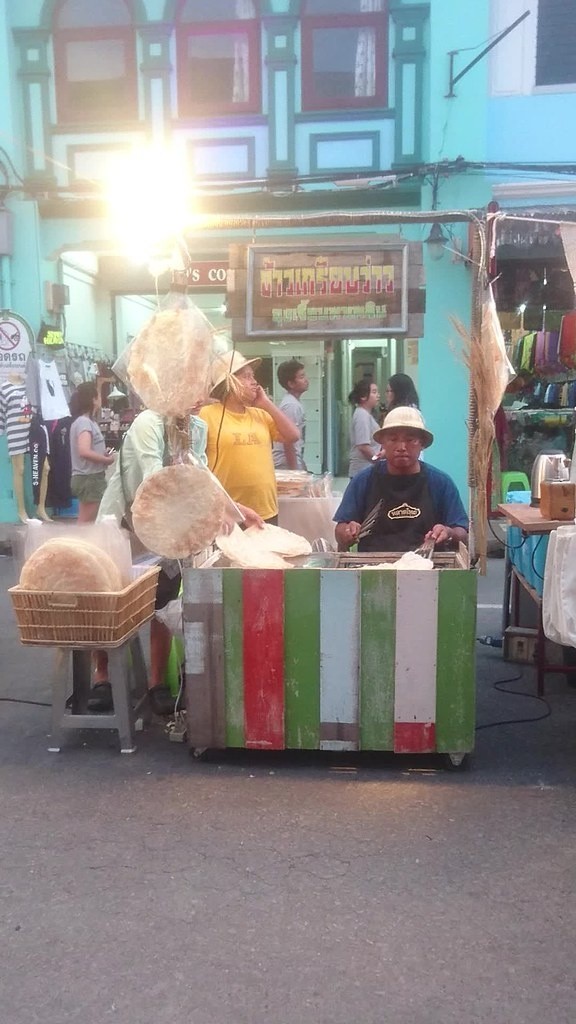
[424,223,448,262]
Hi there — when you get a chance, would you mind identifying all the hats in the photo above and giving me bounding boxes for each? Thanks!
[372,405,434,451]
[207,349,262,399]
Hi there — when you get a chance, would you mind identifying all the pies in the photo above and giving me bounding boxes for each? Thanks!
[215,519,313,570]
[361,551,434,570]
[17,538,121,592]
[128,308,210,418]
[131,464,226,559]
[275,469,333,499]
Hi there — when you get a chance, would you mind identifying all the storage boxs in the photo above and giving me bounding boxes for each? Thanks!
[540,479,576,520]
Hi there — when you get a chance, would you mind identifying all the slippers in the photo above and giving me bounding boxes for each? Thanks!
[87,680,113,711]
[148,684,176,715]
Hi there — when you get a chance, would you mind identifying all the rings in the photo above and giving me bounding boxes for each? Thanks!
[345,529,350,532]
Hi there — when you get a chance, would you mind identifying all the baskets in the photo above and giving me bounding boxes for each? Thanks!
[8,563,163,649]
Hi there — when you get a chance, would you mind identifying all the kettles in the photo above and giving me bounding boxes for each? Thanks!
[530,448,568,507]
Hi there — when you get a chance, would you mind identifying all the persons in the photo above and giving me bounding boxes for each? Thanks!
[197,349,300,530]
[69,381,118,525]
[331,406,469,553]
[347,380,382,482]
[88,390,266,717]
[271,359,309,471]
[376,373,425,460]
[0,371,54,524]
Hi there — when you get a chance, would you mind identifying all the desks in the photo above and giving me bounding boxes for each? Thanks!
[278,492,344,548]
[497,504,576,696]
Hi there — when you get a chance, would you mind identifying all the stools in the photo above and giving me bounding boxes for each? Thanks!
[500,470,530,504]
[49,646,148,754]
